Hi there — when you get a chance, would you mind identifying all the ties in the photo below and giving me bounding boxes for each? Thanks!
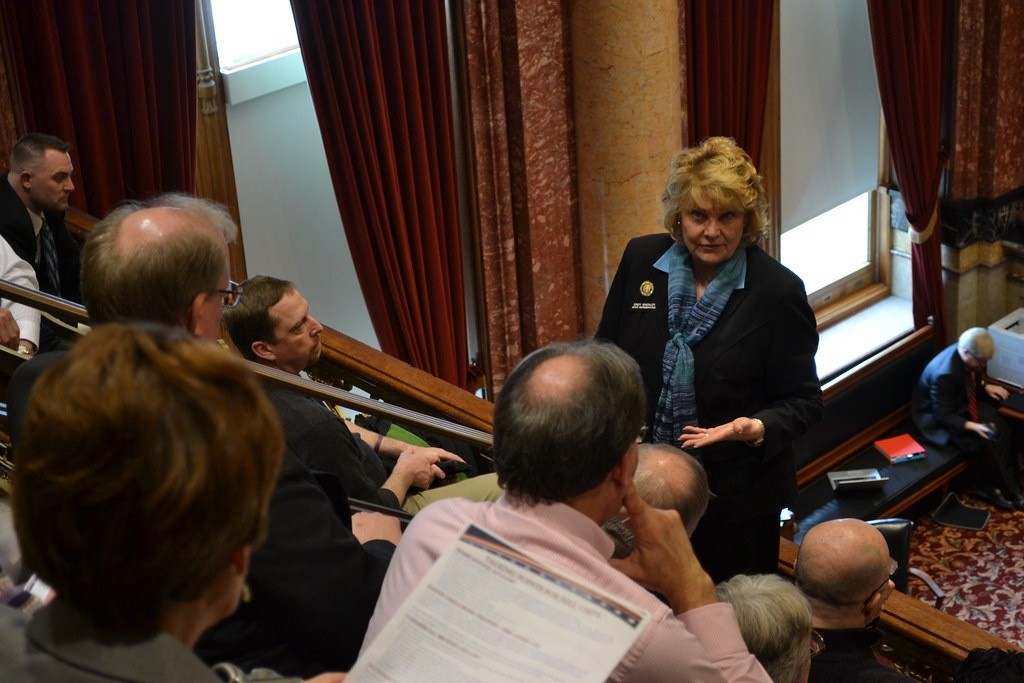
[964,363,979,425]
[41,219,64,299]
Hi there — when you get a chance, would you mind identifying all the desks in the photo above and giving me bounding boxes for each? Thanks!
[981,371,1024,420]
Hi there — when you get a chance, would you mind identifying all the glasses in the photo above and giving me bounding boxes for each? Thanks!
[864,557,899,605]
[636,422,650,444]
[808,629,826,660]
[214,279,245,308]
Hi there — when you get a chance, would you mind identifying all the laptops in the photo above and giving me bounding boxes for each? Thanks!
[827,467,889,491]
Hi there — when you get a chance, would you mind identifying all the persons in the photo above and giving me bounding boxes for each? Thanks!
[3,135,1023,683]
[914,327,1024,513]
[590,135,822,588]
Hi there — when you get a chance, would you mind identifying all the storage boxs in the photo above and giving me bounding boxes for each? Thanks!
[986,305,1024,388]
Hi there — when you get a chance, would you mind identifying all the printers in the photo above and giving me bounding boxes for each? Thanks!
[985,307,1024,389]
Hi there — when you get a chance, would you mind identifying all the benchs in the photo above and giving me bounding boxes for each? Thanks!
[793,426,966,544]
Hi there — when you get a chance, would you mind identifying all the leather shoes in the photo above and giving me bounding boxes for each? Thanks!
[973,472,1024,511]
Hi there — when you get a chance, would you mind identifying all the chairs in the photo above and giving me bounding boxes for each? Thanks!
[863,517,946,608]
